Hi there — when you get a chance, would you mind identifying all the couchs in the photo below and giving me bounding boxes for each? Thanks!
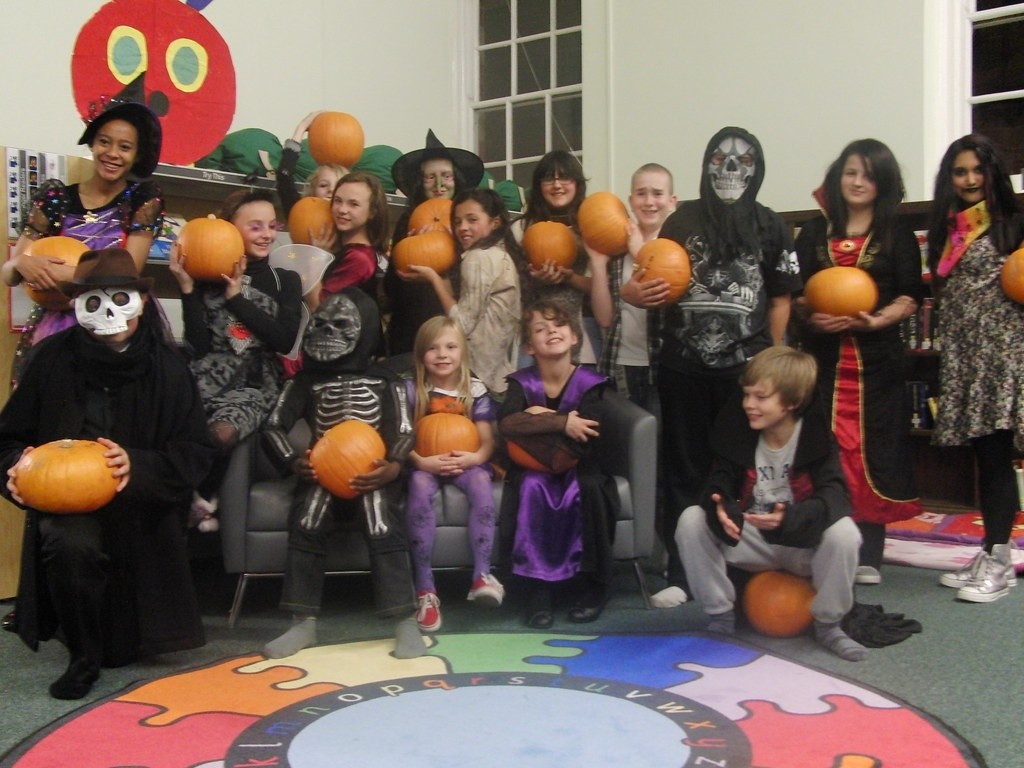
[214,393,663,629]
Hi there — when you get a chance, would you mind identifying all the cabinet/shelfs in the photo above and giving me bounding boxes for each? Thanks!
[66,155,523,299]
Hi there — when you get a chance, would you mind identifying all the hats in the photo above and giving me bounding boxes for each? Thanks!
[392,129,485,200]
[57,248,154,299]
[77,72,162,179]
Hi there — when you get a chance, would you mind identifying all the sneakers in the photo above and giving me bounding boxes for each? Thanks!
[855,565,881,584]
[466,572,506,608]
[416,590,441,632]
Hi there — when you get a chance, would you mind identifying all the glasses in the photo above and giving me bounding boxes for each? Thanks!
[539,172,575,184]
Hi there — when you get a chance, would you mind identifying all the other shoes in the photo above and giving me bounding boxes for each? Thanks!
[1,611,16,628]
[50,663,93,699]
[649,585,688,608]
[186,487,219,531]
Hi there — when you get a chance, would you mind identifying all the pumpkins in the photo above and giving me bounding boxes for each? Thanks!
[414,412,479,457]
[308,420,386,500]
[742,569,816,638]
[287,196,336,246]
[21,235,92,310]
[506,439,579,473]
[307,110,365,167]
[392,231,458,275]
[802,265,878,317]
[631,239,691,305]
[176,214,245,281]
[523,220,579,271]
[14,438,122,516]
[407,197,453,234]
[1000,246,1024,307]
[578,192,631,256]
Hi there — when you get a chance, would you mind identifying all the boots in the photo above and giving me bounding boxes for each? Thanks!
[939,537,1018,588]
[957,544,1009,603]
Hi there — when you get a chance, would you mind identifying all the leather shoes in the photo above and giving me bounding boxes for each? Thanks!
[569,598,602,624]
[527,603,554,629]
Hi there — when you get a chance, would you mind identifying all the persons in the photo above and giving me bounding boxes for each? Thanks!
[673,345,867,660]
[584,162,677,412]
[496,291,622,628]
[307,170,392,312]
[926,134,1024,602]
[395,188,524,393]
[618,126,804,607]
[0,103,167,396]
[384,128,484,352]
[0,248,201,700]
[277,111,351,221]
[403,316,506,631]
[170,189,302,531]
[788,138,925,584]
[259,287,427,658]
[509,149,603,369]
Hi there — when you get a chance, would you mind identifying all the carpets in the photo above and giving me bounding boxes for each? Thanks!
[0,626,988,768]
